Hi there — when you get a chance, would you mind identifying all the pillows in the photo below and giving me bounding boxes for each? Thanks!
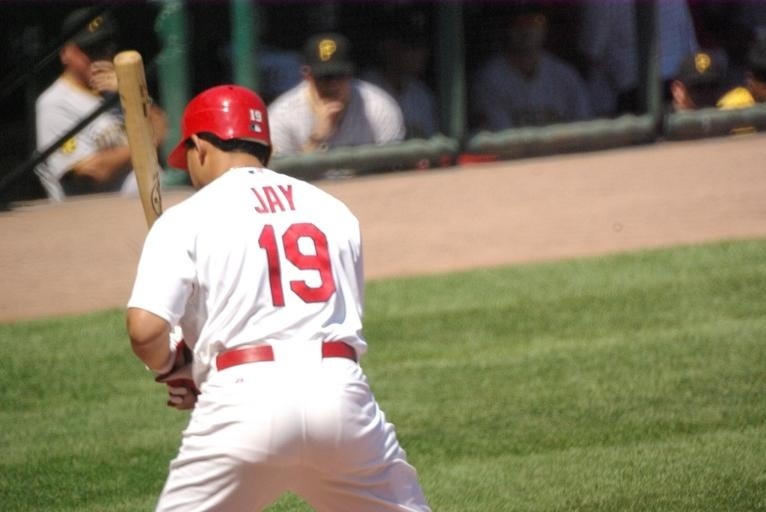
[167,84,274,172]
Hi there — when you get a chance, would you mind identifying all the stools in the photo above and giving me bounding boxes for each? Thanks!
[214,340,360,372]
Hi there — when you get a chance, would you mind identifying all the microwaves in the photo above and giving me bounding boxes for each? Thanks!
[113,50,198,413]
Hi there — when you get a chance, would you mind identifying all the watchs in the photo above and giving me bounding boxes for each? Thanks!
[308,132,333,152]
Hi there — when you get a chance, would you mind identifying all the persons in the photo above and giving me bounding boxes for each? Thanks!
[259,8,306,103]
[468,1,605,164]
[660,45,729,136]
[291,1,364,79]
[714,42,765,134]
[365,26,438,140]
[579,1,701,113]
[728,1,765,57]
[266,32,405,180]
[35,5,166,196]
[128,86,434,511]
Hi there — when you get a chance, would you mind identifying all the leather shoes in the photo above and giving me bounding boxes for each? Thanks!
[143,338,201,412]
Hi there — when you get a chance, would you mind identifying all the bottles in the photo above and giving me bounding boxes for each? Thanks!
[677,46,733,90]
[295,30,365,78]
[59,5,120,49]
